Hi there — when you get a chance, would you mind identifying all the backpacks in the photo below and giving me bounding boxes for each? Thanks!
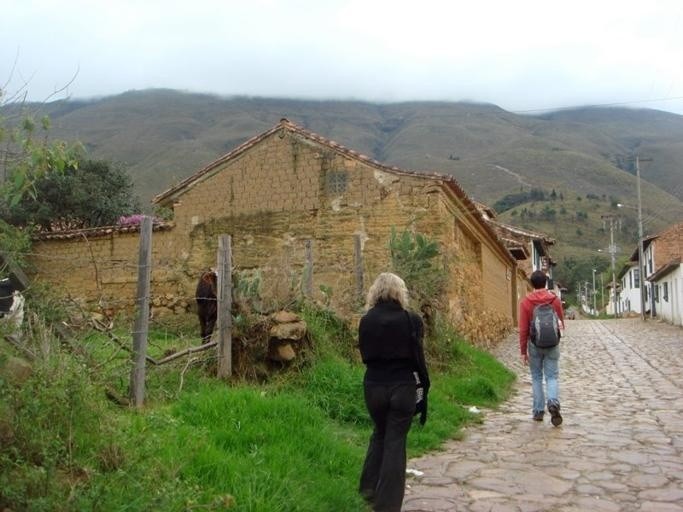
[529,303,561,349]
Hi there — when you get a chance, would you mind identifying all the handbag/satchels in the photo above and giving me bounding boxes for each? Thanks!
[411,361,430,426]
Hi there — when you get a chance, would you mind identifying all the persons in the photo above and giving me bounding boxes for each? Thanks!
[231,302,247,325]
[518,270,566,428]
[356,270,429,511]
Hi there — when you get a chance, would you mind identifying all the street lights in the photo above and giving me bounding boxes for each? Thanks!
[593,203,646,317]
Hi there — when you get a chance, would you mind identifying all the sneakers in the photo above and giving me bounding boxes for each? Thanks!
[532,412,544,421]
[548,404,562,426]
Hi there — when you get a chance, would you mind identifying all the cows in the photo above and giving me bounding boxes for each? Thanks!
[196,270,218,346]
[0,273,26,337]
